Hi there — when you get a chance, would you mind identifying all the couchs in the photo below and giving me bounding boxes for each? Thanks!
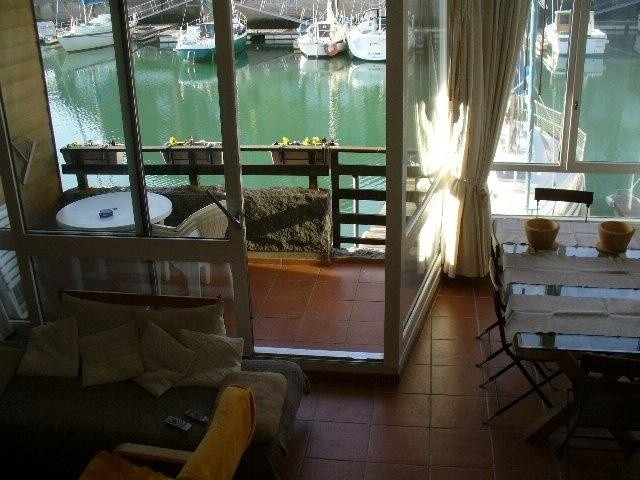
[0,291,308,480]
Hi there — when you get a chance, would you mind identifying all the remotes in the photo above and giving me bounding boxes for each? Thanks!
[164,416,193,432]
[184,407,209,423]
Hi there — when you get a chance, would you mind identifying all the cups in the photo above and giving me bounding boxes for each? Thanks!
[524,218,560,250]
[599,220,635,251]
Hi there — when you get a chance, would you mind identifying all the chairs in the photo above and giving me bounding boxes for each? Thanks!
[558,348,640,461]
[154,199,239,303]
[78,384,259,480]
[534,188,595,221]
[486,257,565,425]
[472,236,558,391]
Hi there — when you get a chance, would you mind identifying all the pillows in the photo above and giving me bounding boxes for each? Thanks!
[135,320,197,396]
[176,328,245,389]
[56,293,150,336]
[16,315,82,380]
[129,301,228,337]
[76,319,146,388]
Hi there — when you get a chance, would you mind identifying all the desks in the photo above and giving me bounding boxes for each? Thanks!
[490,215,640,450]
[56,191,174,290]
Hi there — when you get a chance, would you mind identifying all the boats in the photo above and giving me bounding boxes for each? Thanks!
[348,0,416,60]
[537,50,605,86]
[176,49,249,85]
[346,62,417,91]
[173,0,249,65]
[547,8,609,56]
[295,0,355,61]
[60,39,137,75]
[427,78,589,225]
[297,51,349,78]
[56,1,139,53]
